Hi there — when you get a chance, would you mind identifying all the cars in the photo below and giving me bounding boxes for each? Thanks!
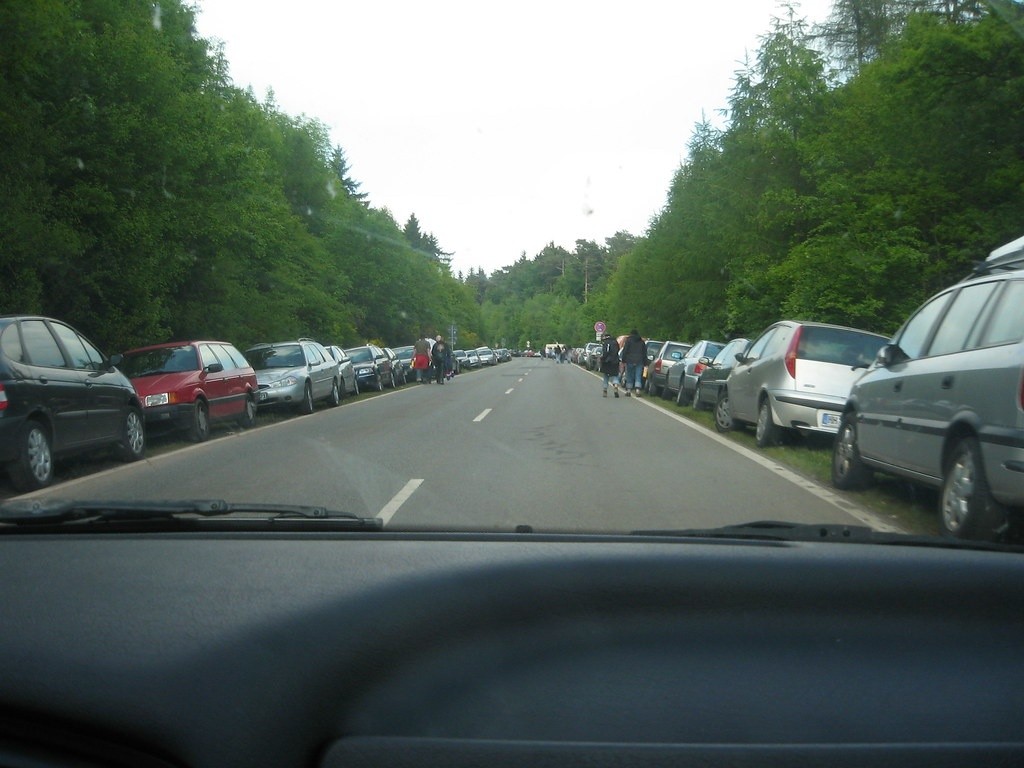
[0,316,148,493]
[382,347,406,386]
[664,340,724,407]
[392,346,417,380]
[692,338,751,410]
[112,341,260,442]
[453,351,471,370]
[449,349,459,375]
[511,335,663,387]
[325,347,359,398]
[478,348,511,365]
[645,341,692,394]
[712,321,892,448]
[464,350,482,368]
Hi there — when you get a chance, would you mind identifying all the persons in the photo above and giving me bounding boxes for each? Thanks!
[601,335,620,398]
[622,329,648,397]
[554,345,572,364]
[540,347,552,360]
[413,334,432,384]
[432,336,447,384]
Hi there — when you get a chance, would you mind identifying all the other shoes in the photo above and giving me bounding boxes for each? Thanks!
[603,390,607,397]
[636,392,641,397]
[614,390,619,398]
[625,392,630,396]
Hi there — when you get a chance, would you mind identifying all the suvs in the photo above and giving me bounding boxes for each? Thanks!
[344,344,396,391]
[832,234,1023,535]
[241,338,341,415]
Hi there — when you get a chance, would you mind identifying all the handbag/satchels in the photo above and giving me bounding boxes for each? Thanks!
[601,351,618,366]
